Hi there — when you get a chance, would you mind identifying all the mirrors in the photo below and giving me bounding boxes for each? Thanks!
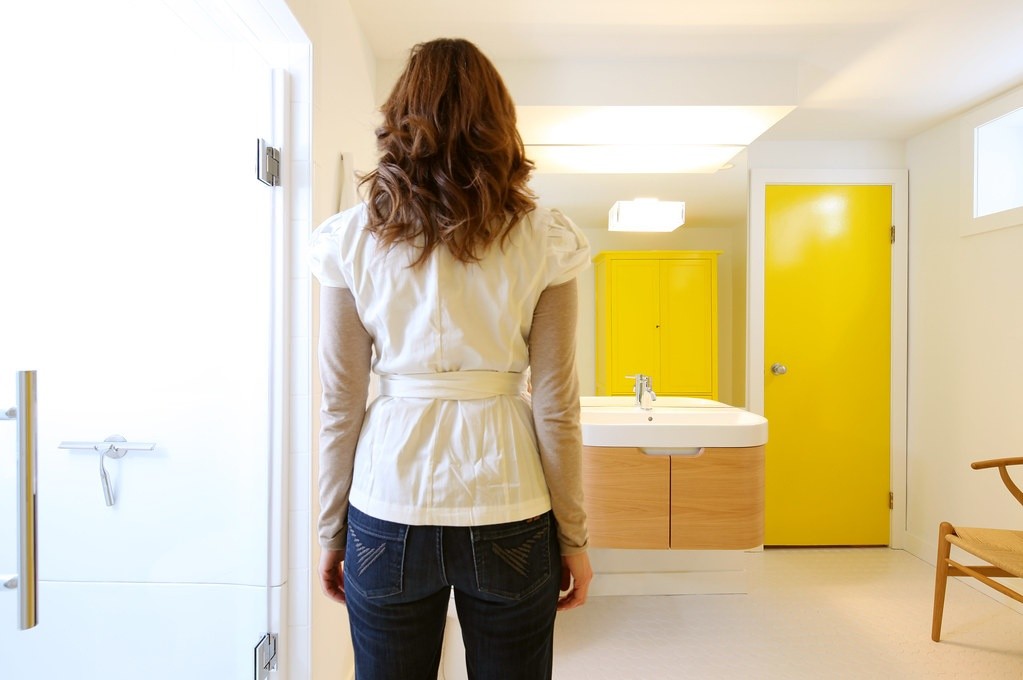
[518,142,750,408]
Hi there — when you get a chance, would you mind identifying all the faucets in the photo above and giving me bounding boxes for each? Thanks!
[624,375,643,405]
[641,375,656,407]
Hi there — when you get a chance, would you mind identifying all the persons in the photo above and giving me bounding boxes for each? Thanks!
[305,38,592,680]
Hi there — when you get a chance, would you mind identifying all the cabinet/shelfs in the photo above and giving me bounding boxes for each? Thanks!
[582,445,766,551]
[590,250,719,399]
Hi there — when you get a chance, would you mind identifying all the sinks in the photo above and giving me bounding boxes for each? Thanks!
[580,398,732,408]
[579,408,767,448]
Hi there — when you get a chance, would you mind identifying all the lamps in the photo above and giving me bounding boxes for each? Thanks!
[608,197,686,233]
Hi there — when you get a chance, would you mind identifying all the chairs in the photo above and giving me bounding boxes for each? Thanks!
[932,456,1023,642]
